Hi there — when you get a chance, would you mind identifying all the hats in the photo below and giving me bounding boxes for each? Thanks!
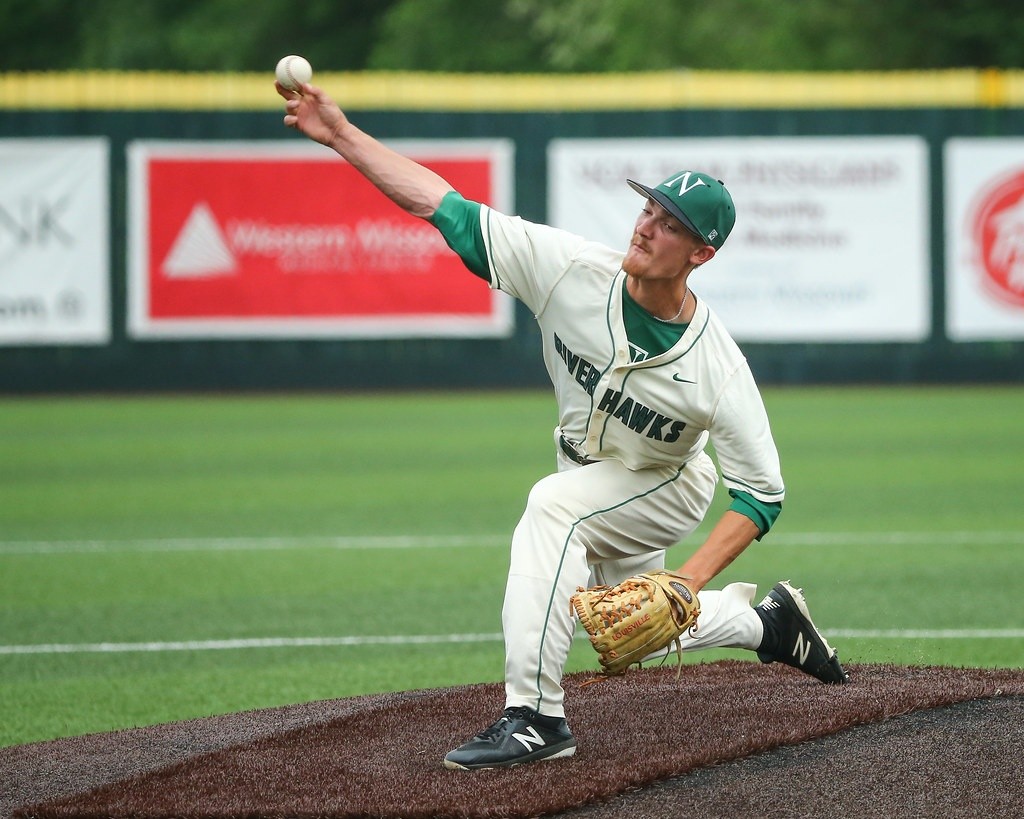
[626,170,736,252]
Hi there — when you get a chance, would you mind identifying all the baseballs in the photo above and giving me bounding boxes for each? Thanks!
[274,54,313,93]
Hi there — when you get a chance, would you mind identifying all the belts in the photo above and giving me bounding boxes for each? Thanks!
[559,435,600,467]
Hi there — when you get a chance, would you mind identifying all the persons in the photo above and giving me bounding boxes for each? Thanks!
[273,76,852,770]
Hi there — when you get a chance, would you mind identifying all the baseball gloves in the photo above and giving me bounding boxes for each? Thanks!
[570,570,703,675]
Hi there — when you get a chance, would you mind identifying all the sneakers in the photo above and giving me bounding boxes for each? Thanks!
[444,705,577,769]
[756,580,848,685]
